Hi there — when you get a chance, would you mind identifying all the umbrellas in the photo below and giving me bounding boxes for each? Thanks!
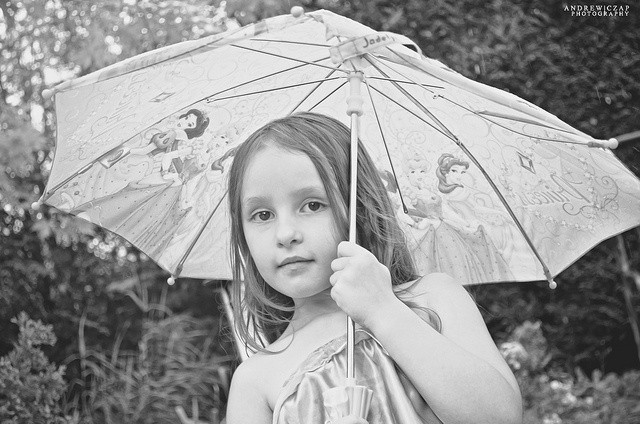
[32,5,640,424]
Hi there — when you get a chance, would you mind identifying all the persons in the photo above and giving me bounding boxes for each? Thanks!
[221,112,522,424]
